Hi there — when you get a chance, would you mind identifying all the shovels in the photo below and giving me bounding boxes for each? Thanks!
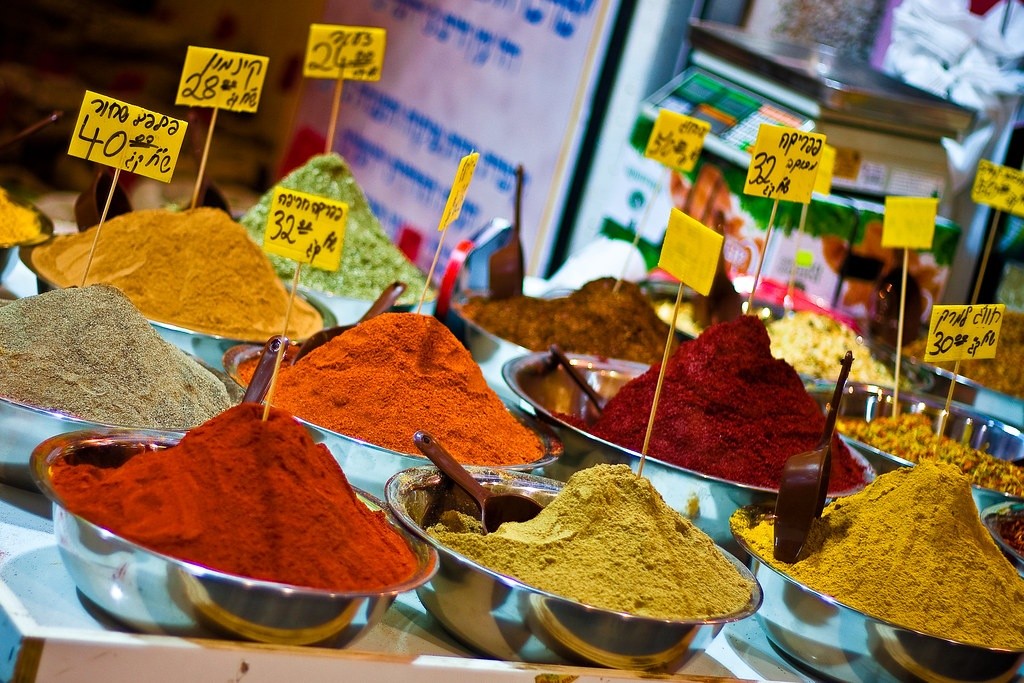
[176,182,233,230]
[769,347,856,565]
[548,343,607,412]
[487,167,525,296]
[291,281,409,384]
[410,429,546,535]
[0,104,70,162]
[73,169,136,232]
[239,334,291,404]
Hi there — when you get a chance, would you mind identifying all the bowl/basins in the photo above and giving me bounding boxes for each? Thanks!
[0,350,244,494]
[888,348,1024,429]
[729,503,1024,683]
[21,235,338,371]
[385,466,764,674]
[981,501,1024,578]
[804,386,1024,477]
[637,281,934,393]
[30,426,440,648]
[502,354,879,566]
[221,342,563,502]
[449,293,539,413]
[289,284,440,326]
[0,189,52,284]
